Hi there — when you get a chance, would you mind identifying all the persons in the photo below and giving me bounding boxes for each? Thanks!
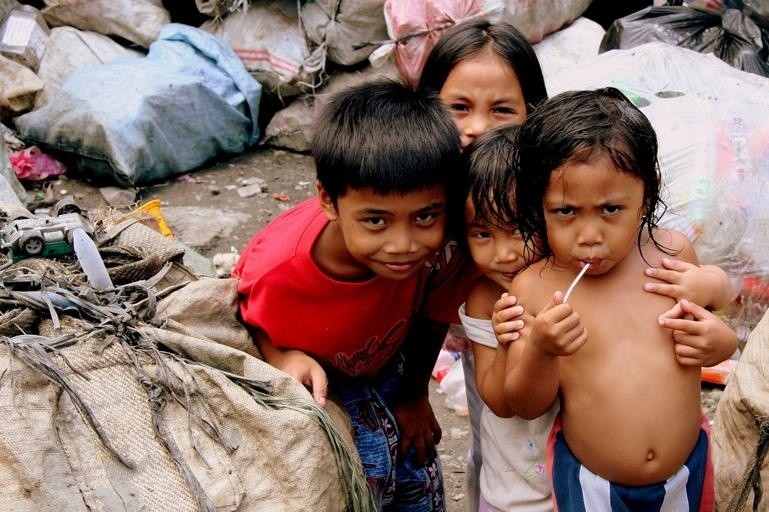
[459,123,732,512]
[502,85,741,512]
[228,74,466,512]
[417,19,549,512]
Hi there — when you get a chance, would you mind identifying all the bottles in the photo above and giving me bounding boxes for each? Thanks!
[433,348,471,417]
